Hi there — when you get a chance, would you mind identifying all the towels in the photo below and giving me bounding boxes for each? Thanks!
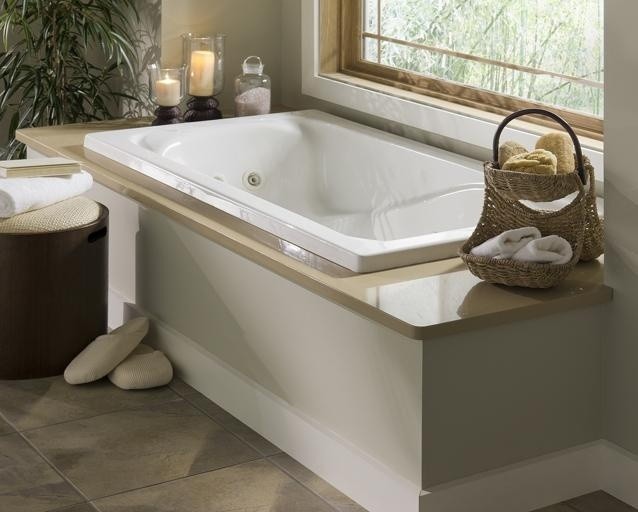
[469,226,542,260]
[0,166,94,219]
[513,234,574,264]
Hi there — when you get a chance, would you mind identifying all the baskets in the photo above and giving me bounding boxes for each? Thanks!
[457,153,604,288]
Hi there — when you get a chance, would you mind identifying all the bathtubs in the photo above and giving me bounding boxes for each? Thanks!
[83,107,607,274]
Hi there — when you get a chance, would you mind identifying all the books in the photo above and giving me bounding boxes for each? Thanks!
[0,157,82,178]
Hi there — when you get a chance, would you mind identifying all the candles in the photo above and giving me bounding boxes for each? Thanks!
[157,75,180,106]
[188,51,214,96]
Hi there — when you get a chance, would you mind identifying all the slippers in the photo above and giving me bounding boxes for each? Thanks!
[63,317,173,390]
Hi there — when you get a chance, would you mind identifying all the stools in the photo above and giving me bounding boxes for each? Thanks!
[0,194,109,379]
